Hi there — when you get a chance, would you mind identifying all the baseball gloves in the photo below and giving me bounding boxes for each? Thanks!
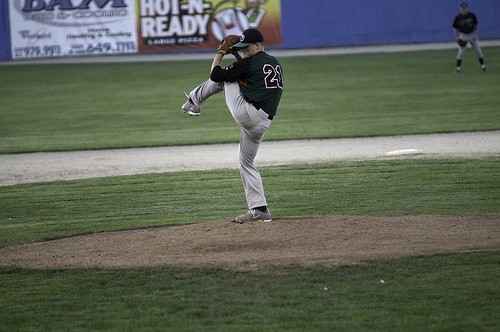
[457,38,466,47]
[216,35,242,54]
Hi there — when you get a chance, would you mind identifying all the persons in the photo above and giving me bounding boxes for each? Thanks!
[181,28,284,224]
[452,2,487,72]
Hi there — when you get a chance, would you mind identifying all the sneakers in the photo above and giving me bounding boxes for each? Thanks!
[182,92,200,116]
[235,208,272,223]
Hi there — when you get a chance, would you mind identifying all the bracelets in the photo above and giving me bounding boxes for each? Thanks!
[217,52,224,57]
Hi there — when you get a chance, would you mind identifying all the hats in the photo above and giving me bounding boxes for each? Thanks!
[460,3,468,8]
[233,28,264,48]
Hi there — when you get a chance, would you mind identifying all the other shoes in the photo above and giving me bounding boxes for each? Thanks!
[456,66,461,72]
[480,65,486,70]
